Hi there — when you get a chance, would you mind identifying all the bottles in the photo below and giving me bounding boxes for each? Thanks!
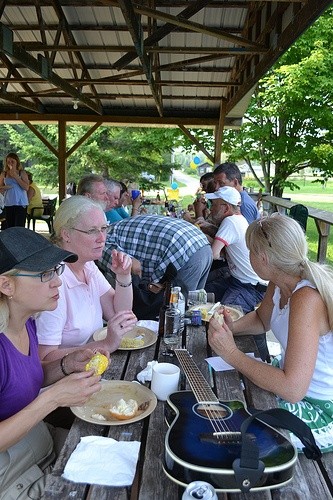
[174,286,185,332]
[164,288,181,345]
[158,284,174,336]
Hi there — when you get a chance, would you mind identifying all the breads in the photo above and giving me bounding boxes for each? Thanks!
[212,312,223,327]
[108,398,138,420]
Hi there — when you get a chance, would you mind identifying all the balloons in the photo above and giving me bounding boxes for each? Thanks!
[171,182,178,190]
[190,157,200,169]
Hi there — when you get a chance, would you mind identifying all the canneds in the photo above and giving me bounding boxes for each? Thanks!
[182,481,219,500]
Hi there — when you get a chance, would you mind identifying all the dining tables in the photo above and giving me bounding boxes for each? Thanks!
[41,306,333,500]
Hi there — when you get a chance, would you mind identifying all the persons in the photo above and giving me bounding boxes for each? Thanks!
[35,196,137,428]
[0,227,112,500]
[77,162,270,319]
[0,153,44,228]
[208,212,333,454]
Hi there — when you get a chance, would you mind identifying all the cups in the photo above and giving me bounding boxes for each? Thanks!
[151,363,180,401]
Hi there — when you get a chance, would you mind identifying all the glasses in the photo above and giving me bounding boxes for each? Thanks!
[10,263,65,283]
[257,212,281,247]
[211,202,226,206]
[69,225,110,238]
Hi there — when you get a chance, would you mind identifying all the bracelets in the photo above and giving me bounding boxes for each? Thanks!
[60,355,70,376]
[115,276,133,288]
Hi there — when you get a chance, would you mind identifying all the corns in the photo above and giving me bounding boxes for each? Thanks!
[121,338,144,348]
[85,352,108,376]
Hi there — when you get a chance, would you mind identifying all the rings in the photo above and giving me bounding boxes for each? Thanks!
[119,322,124,328]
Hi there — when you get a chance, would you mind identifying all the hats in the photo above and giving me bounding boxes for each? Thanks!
[28,175,33,182]
[0,227,79,275]
[204,185,241,206]
[117,181,127,198]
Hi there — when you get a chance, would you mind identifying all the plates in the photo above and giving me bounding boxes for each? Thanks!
[186,304,243,324]
[93,325,157,350]
[70,380,157,426]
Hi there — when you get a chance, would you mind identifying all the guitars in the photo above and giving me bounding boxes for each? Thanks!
[161,338,299,495]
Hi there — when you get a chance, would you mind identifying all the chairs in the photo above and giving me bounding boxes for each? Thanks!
[26,197,58,234]
